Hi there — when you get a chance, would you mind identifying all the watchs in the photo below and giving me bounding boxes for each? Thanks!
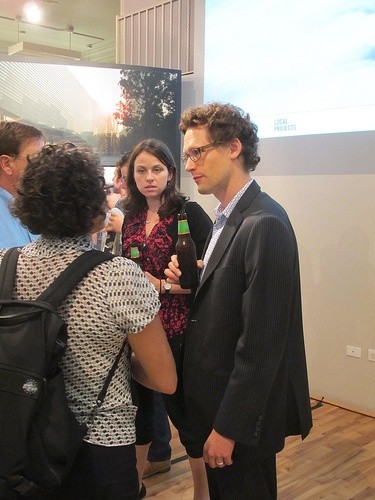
[164,279,172,295]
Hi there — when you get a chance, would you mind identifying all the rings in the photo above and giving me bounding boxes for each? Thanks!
[216,462,224,465]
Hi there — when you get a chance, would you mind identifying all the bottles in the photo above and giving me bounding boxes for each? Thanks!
[175,212,200,289]
[129,243,145,273]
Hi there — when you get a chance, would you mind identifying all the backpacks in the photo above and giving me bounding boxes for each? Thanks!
[0,246,129,500]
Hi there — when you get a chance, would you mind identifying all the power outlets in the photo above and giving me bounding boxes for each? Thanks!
[346,346,362,358]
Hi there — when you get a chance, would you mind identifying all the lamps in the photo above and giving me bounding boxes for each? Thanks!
[8,15,81,62]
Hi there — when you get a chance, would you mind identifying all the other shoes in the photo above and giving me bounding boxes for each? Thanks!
[139,482,147,498]
[142,459,171,479]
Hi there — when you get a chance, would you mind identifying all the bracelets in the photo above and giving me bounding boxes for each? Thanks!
[160,278,161,295]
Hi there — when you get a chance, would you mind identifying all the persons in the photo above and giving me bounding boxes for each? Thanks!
[0,141,178,500]
[0,120,50,254]
[178,101,313,500]
[87,149,133,257]
[121,138,213,500]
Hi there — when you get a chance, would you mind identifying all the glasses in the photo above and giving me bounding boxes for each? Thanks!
[96,176,105,189]
[180,141,221,164]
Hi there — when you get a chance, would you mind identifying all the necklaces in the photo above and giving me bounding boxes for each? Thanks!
[145,205,161,224]
